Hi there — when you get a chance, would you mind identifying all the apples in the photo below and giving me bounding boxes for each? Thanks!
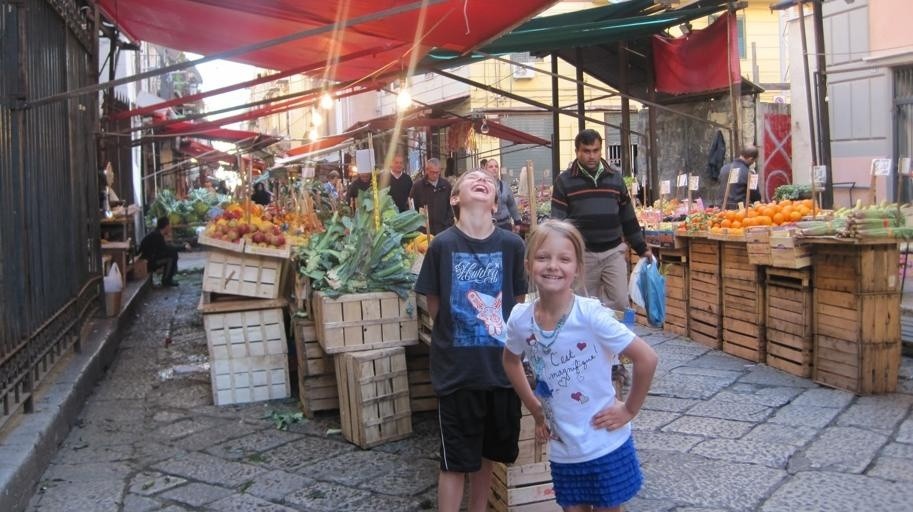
[205,219,286,249]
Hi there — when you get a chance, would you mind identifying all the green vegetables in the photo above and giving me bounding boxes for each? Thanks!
[794,209,913,240]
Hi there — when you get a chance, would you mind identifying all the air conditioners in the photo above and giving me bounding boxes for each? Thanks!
[512,62,536,79]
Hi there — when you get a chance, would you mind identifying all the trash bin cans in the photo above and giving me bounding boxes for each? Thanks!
[101,276,122,317]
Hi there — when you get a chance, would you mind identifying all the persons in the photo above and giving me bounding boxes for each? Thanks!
[137,216,191,289]
[713,144,763,208]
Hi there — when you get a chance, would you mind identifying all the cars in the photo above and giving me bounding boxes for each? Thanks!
[195,174,220,191]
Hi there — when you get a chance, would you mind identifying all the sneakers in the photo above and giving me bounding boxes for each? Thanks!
[161,279,180,287]
